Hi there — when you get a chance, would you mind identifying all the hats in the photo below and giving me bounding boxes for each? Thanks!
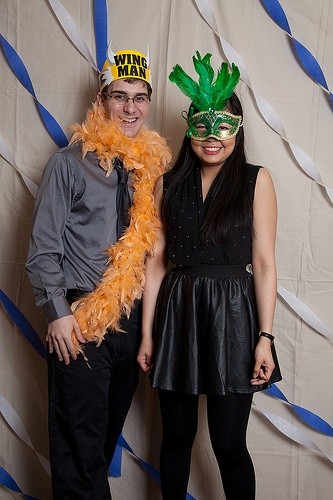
[98,40,153,95]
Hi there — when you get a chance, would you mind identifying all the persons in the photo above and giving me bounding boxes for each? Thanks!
[134,86,278,500]
[26,39,174,500]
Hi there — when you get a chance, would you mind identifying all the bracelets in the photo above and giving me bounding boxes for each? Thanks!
[259,331,275,346]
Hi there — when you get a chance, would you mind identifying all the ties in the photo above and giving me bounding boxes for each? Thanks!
[112,157,132,243]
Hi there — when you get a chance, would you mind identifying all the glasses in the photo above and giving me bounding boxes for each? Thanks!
[101,90,150,107]
